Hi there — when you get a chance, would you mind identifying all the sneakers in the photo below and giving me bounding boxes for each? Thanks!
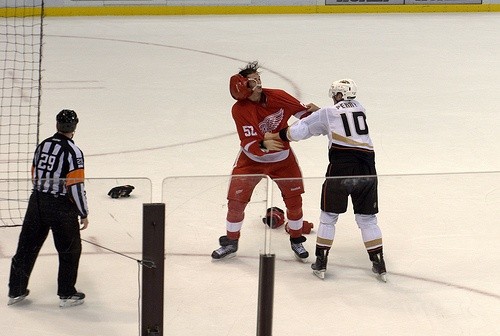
[310,244,331,279]
[369,249,388,282]
[290,237,309,262]
[58,291,85,308]
[211,237,239,261]
[7,288,30,306]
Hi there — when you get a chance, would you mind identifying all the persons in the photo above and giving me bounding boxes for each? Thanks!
[211,60,313,262]
[5,109,90,310]
[264,79,388,284]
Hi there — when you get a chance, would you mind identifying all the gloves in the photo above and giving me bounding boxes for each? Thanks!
[262,207,284,228]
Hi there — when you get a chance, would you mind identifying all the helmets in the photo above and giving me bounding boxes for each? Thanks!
[56,109,78,133]
[329,79,357,101]
[230,75,258,99]
